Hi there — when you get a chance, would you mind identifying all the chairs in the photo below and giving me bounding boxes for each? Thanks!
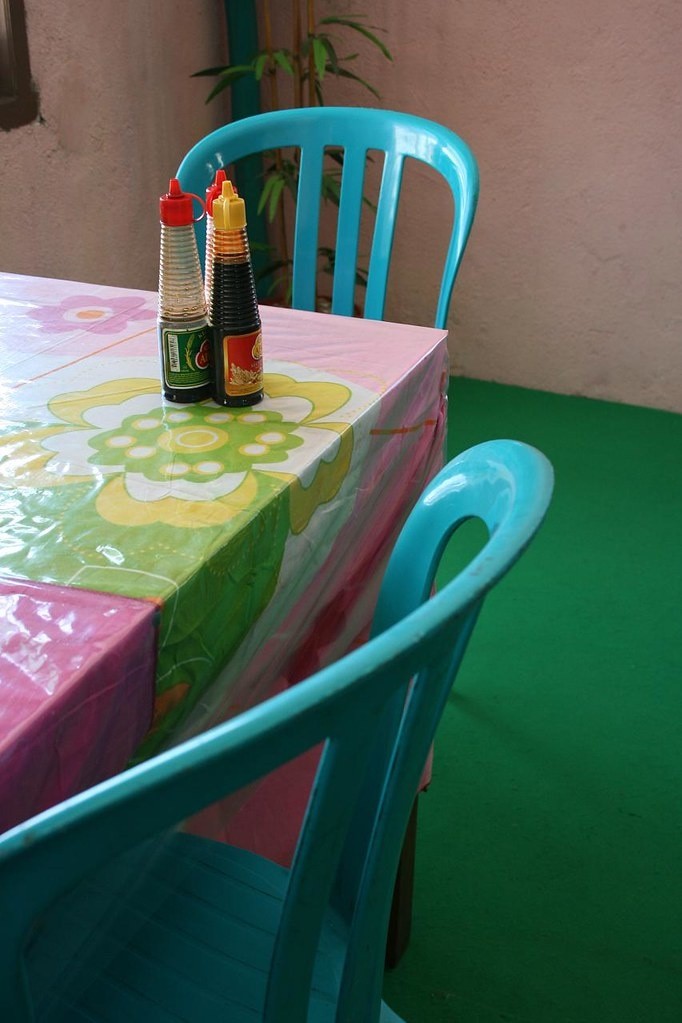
[176,105,479,330]
[0,440,554,1023]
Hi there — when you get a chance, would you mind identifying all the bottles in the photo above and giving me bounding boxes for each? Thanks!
[209,180,265,408]
[205,170,238,304]
[156,178,211,404]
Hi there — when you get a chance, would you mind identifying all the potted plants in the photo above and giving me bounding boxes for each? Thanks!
[189,0,393,319]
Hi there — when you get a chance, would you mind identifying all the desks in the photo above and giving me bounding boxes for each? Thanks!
[0,273,450,973]
